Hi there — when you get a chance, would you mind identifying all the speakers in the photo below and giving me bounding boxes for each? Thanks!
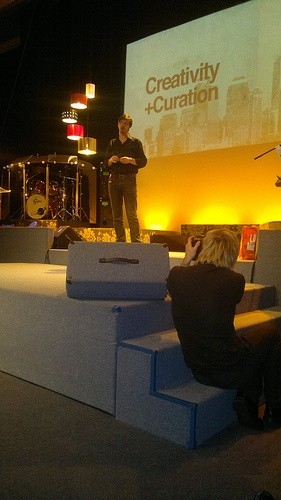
[66,241,170,300]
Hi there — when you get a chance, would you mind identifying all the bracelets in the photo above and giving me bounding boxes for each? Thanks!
[129,157,132,164]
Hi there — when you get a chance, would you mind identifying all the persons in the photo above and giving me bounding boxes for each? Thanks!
[167,227,281,434]
[101,113,148,243]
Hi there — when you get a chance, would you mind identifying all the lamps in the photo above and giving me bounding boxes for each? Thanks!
[61,48,97,156]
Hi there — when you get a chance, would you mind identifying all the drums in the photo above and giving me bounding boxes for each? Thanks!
[26,179,45,194]
[25,193,58,220]
[44,180,59,197]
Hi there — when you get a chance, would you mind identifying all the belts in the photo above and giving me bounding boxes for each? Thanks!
[111,174,136,178]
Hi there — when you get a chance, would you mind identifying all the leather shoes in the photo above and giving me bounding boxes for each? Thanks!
[263,407,281,427]
[232,392,264,430]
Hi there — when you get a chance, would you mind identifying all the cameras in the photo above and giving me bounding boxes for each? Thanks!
[191,235,206,261]
